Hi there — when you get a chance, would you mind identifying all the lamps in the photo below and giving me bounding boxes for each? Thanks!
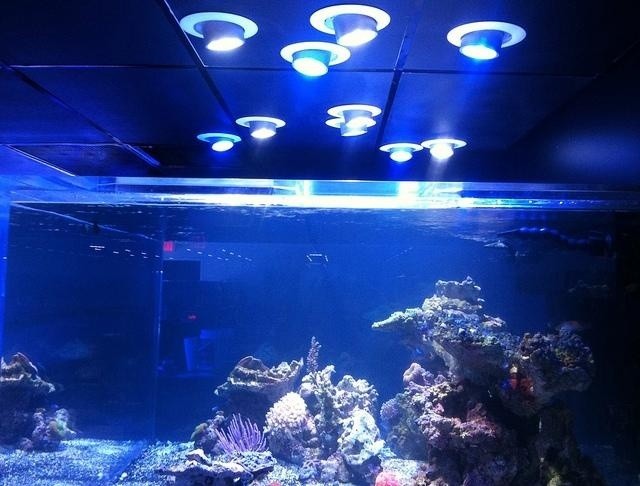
[429,142,455,161]
[202,21,248,54]
[338,122,368,137]
[331,11,380,47]
[341,110,377,129]
[209,136,235,152]
[248,121,278,139]
[459,30,504,61]
[389,146,415,164]
[290,48,331,78]
